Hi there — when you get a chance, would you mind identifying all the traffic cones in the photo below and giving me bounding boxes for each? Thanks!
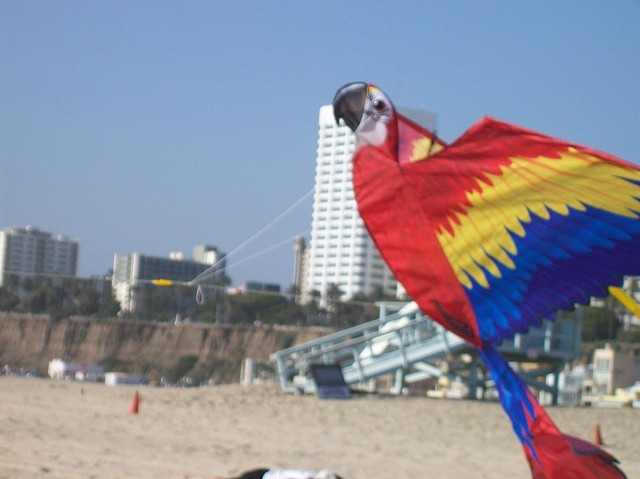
[131,390,139,414]
[593,423,603,445]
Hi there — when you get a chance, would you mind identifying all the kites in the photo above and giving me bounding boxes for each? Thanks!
[329,79,640,478]
